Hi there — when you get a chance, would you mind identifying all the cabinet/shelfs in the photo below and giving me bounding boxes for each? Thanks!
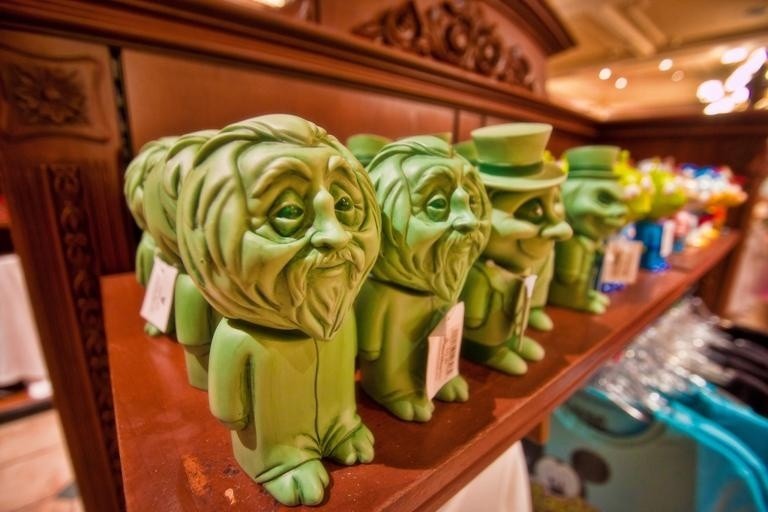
[0,0,768,512]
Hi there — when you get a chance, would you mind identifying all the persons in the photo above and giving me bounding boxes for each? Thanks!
[173,113,375,506]
[549,145,630,315]
[527,249,557,331]
[345,134,392,166]
[469,121,574,377]
[144,128,224,391]
[122,134,179,338]
[355,134,494,422]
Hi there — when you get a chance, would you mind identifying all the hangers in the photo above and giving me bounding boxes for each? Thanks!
[586,291,728,423]
